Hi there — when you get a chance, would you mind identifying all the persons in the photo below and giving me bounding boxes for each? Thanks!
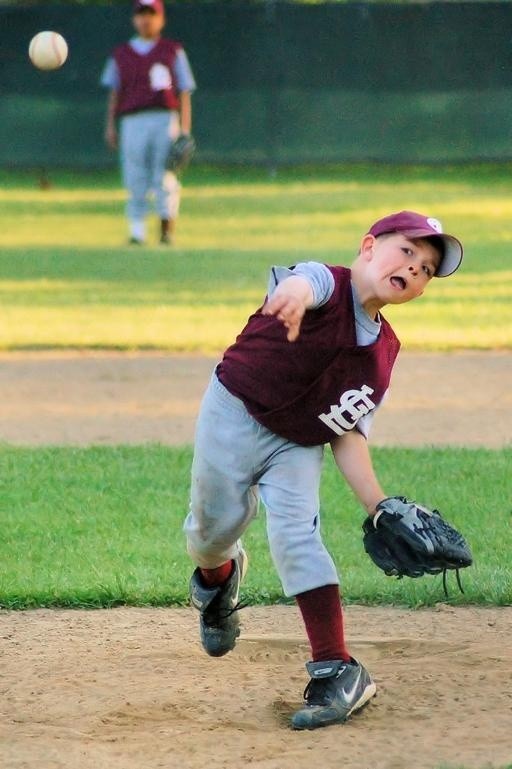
[100,0,198,247]
[182,210,470,730]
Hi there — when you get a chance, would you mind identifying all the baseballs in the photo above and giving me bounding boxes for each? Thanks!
[27,31,68,71]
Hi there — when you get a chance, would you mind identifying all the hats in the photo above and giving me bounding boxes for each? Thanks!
[369,210,464,278]
[134,0,164,15]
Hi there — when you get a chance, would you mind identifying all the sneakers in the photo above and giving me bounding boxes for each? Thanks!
[190,548,248,657]
[291,656,377,729]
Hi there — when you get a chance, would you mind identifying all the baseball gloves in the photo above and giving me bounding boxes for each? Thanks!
[166,136,195,172]
[363,495,472,578]
